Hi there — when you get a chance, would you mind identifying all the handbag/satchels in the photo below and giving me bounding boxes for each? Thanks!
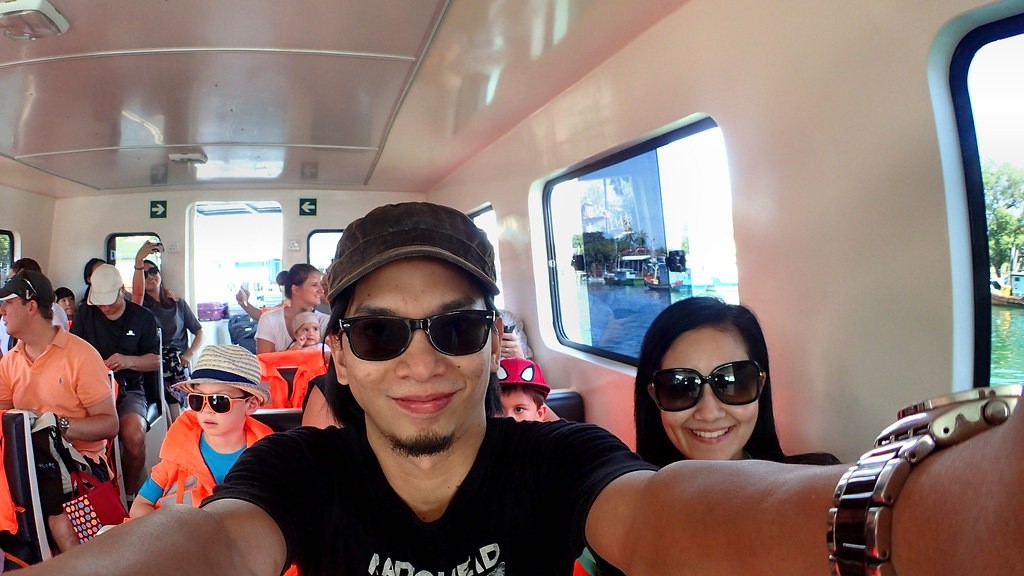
[63,471,130,544]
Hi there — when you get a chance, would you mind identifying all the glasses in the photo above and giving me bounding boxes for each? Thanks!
[16,269,39,297]
[144,267,157,278]
[187,393,247,413]
[339,310,496,361]
[648,360,763,412]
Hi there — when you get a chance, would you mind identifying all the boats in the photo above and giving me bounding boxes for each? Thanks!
[604,268,644,287]
[643,270,683,290]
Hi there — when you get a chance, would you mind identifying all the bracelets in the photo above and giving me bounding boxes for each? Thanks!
[134,266,144,270]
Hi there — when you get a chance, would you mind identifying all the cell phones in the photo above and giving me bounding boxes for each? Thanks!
[149,241,165,251]
[501,323,517,340]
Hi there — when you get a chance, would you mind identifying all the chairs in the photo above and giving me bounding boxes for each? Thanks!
[0,328,584,563]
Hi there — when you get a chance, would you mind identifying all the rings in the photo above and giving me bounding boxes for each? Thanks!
[505,347,508,353]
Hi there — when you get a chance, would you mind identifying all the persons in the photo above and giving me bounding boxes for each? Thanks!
[234,261,334,353]
[0,201,1024,576]
[582,232,638,348]
[0,270,121,554]
[52,287,75,330]
[69,264,162,510]
[497,307,536,362]
[128,240,204,405]
[76,258,107,310]
[0,257,69,361]
[301,355,367,429]
[628,296,842,473]
[492,356,551,421]
[126,345,278,521]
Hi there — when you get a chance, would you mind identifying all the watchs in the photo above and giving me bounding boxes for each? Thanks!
[825,382,1020,575]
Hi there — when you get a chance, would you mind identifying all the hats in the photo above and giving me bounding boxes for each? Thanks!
[0,270,55,308]
[171,345,270,407]
[327,201,500,303]
[90,264,123,305]
[496,358,550,403]
[292,312,319,334]
